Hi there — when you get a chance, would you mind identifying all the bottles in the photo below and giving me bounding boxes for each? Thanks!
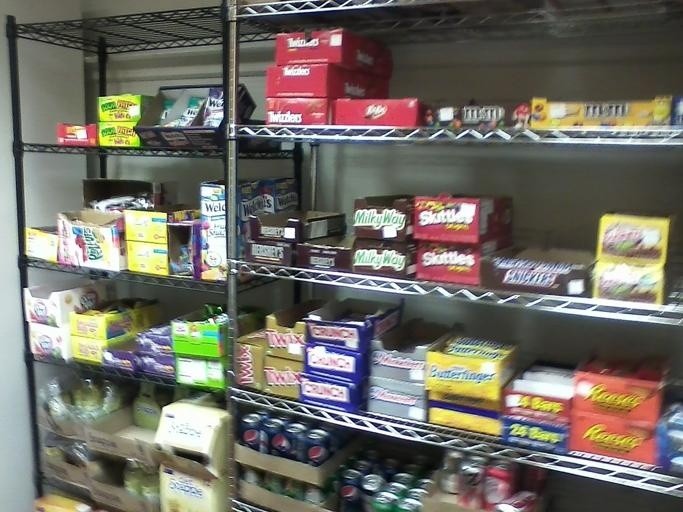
[41,376,163,512]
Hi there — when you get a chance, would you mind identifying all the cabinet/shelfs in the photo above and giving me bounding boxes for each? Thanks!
[1,1,232,512]
[235,0,683,511]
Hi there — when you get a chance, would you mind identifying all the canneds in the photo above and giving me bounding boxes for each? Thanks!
[340,449,437,512]
[239,409,339,466]
[439,450,548,512]
